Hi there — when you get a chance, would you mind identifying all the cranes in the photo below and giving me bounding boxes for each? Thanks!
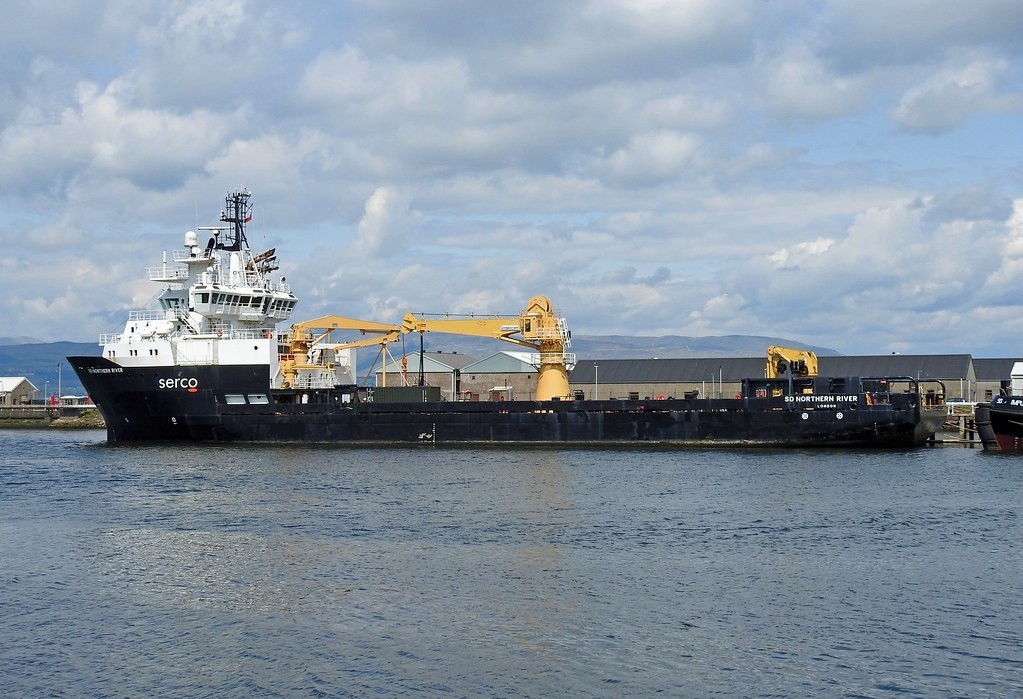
[279,292,579,402]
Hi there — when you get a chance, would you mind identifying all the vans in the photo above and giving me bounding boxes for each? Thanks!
[945,397,966,405]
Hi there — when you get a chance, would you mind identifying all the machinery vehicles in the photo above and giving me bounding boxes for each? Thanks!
[764,345,818,397]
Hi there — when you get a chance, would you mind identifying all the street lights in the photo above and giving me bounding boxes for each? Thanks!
[44,380,50,411]
[0,381,4,406]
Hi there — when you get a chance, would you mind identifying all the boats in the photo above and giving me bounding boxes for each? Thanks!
[65,183,949,453]
[974,394,1023,456]
